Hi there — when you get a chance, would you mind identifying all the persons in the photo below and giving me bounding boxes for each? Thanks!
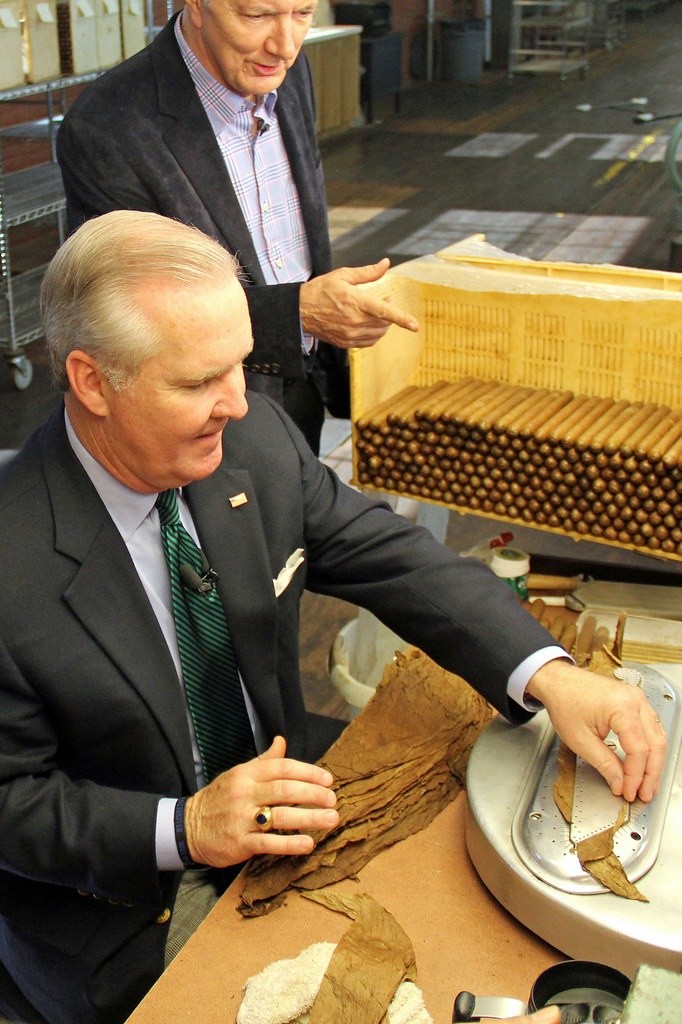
[1,208,674,1024]
[56,2,421,468]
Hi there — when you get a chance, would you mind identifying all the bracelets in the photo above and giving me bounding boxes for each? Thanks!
[174,796,196,866]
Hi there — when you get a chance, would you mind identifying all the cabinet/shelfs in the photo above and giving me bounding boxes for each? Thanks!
[0,66,110,390]
[299,28,364,138]
[507,0,595,85]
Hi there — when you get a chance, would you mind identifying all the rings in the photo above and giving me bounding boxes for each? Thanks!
[254,805,274,832]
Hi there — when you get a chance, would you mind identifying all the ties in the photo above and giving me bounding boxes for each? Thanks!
[155,488,259,785]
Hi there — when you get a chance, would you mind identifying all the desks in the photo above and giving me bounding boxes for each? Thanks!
[127,646,682,1024]
